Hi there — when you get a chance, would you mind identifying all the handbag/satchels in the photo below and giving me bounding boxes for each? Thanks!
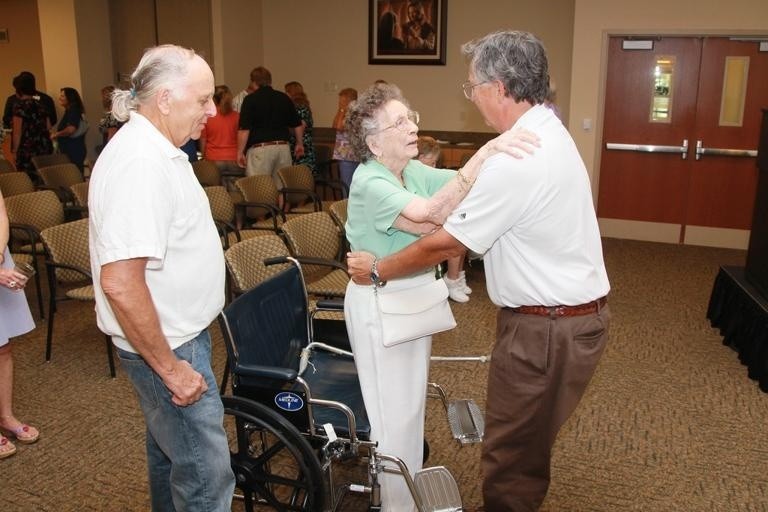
[63,120,91,143]
[106,127,117,142]
[376,277,459,348]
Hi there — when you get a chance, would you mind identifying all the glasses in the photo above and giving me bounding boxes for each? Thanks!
[374,111,420,134]
[462,80,487,99]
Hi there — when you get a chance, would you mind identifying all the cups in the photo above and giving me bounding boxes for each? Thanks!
[8,262,36,293]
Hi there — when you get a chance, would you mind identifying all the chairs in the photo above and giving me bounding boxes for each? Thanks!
[40,217,117,378]
[0,159,16,173]
[283,212,350,305]
[193,159,245,188]
[328,199,348,231]
[38,163,86,220]
[3,189,67,321]
[202,185,280,247]
[279,163,349,212]
[71,181,89,215]
[0,171,36,197]
[219,235,344,399]
[31,153,71,169]
[234,173,318,233]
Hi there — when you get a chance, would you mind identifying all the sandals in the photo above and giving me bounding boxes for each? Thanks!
[0,416,41,443]
[0,436,17,459]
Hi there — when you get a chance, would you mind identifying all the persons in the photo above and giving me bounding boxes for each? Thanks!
[343,81,540,512]
[379,11,405,49]
[180,67,366,200]
[1,72,87,182]
[87,44,236,511]
[402,3,434,49]
[344,30,612,511]
[0,189,41,460]
[412,135,472,302]
[97,85,125,147]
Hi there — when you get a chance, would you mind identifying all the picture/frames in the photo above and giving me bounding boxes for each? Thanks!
[367,1,448,66]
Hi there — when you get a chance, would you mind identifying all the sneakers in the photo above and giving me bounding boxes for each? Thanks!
[444,276,470,303]
[458,270,472,295]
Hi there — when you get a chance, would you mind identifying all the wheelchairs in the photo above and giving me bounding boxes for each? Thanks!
[217,257,485,511]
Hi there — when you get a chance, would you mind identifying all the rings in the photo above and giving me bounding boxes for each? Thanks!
[9,280,17,288]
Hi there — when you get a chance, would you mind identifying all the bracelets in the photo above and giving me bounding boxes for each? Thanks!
[454,168,475,196]
[0,253,5,265]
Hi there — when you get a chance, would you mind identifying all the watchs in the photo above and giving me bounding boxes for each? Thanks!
[369,258,386,288]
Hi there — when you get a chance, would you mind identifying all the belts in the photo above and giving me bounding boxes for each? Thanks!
[501,296,608,317]
[251,141,288,147]
